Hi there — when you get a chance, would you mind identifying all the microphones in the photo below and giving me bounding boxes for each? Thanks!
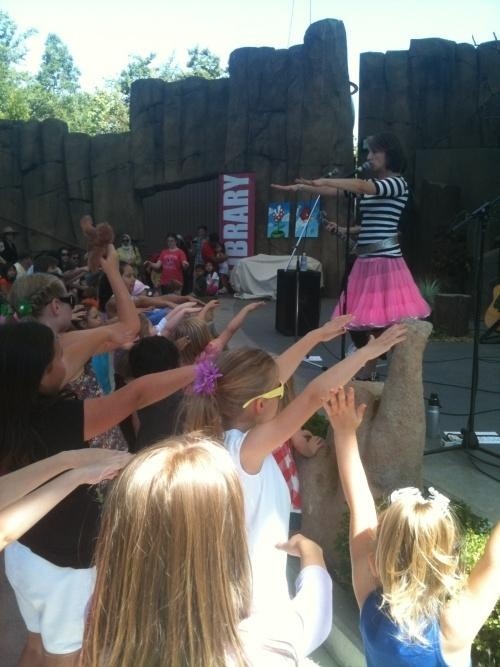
[338,166,364,179]
[324,167,338,177]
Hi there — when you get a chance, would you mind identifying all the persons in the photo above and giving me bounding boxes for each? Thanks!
[267,129,432,381]
[1,210,499,667]
[322,156,415,293]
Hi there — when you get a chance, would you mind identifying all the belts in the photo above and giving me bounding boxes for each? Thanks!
[354,235,399,254]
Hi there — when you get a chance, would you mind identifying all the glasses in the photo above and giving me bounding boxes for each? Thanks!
[62,252,80,259]
[56,293,76,309]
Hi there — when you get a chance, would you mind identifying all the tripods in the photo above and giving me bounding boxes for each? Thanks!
[422,219,500,461]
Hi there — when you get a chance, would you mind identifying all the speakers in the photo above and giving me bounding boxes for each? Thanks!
[275,269,321,336]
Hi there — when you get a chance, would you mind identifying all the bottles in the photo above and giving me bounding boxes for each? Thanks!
[300,252,307,271]
[425,392,441,439]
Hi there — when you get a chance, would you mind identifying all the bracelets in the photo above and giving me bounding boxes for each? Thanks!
[297,182,304,191]
